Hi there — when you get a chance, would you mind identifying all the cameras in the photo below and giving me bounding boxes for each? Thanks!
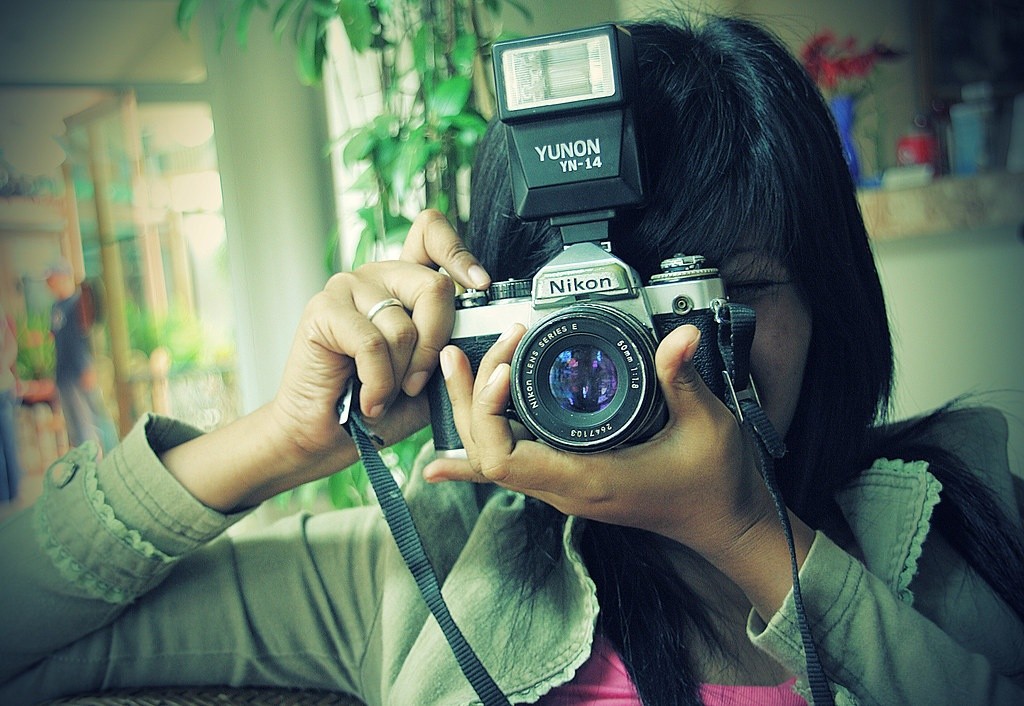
[408,24,730,460]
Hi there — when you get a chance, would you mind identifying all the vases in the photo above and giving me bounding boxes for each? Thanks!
[829,97,860,185]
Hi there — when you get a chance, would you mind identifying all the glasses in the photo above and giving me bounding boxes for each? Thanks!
[734,279,791,317]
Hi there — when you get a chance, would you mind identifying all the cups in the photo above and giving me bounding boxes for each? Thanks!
[897,132,938,179]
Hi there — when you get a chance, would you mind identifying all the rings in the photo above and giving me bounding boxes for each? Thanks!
[367,298,403,321]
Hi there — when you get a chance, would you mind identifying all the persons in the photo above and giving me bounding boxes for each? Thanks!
[0,310,27,502]
[42,257,119,459]
[1,12,1024,706]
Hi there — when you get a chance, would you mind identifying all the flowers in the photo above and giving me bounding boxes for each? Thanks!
[799,30,878,93]
